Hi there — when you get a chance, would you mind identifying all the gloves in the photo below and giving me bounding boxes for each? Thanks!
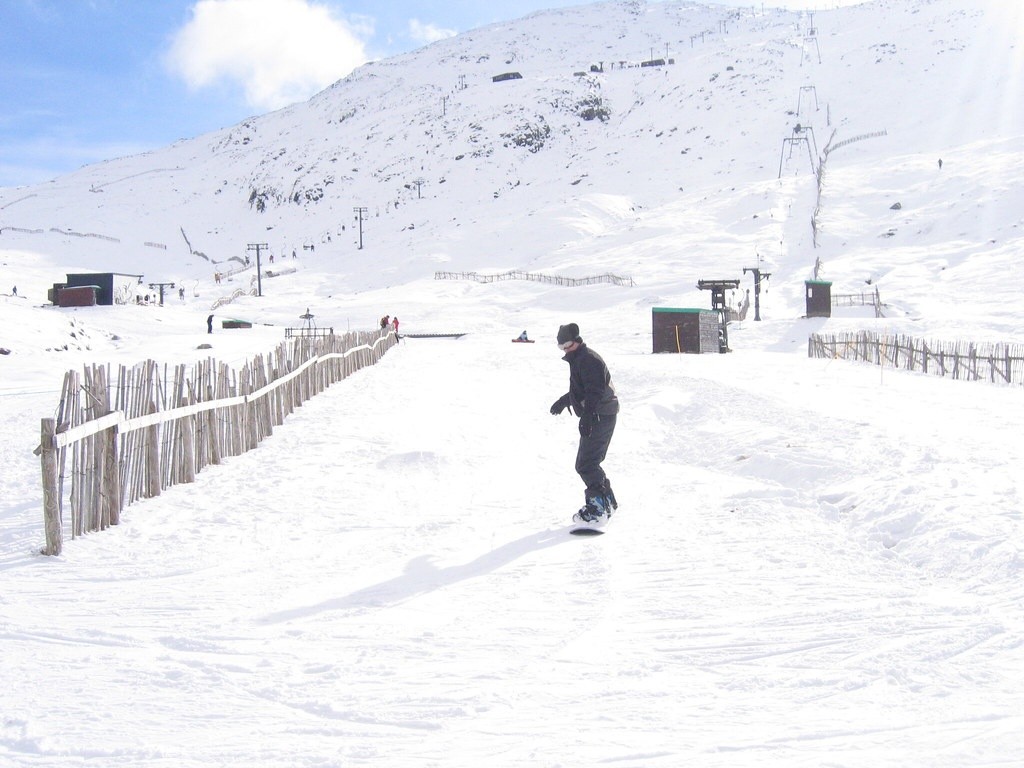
[550,394,573,416]
[579,408,600,438]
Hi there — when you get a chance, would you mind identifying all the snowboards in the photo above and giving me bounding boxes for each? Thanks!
[569,501,616,535]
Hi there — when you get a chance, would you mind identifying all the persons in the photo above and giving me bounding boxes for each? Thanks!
[392,317,399,342]
[179,225,348,305]
[381,315,389,328]
[207,314,214,333]
[550,322,619,522]
[518,330,528,340]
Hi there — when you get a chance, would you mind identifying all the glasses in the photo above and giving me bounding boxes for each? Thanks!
[557,340,574,350]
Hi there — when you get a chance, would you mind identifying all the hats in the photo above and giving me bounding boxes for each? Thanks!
[557,323,579,344]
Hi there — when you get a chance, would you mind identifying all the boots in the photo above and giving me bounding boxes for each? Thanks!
[573,479,618,528]
